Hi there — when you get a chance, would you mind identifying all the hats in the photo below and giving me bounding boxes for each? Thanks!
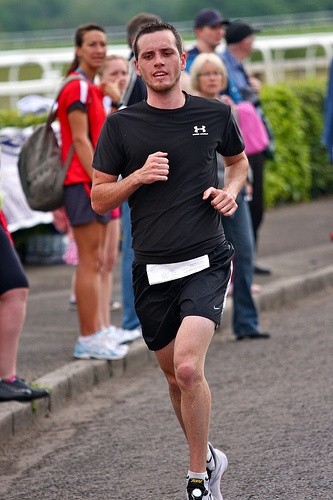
[192,9,231,28]
[224,19,260,43]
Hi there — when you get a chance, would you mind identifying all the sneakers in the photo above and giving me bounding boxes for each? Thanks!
[186,476,216,500]
[73,336,127,360]
[106,326,141,345]
[207,442,229,500]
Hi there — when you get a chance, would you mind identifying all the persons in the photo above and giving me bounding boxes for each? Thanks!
[90,21,251,500]
[0,207,52,402]
[213,19,273,274]
[179,52,271,343]
[115,11,165,114]
[93,54,148,335]
[51,24,141,361]
[52,208,83,305]
[178,7,231,81]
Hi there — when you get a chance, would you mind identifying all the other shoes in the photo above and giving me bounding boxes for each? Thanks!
[0,380,32,402]
[236,332,269,340]
[7,379,49,402]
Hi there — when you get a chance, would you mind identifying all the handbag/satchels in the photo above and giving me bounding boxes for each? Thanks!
[236,100,270,156]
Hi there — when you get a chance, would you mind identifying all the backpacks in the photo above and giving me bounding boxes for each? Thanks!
[17,76,91,211]
